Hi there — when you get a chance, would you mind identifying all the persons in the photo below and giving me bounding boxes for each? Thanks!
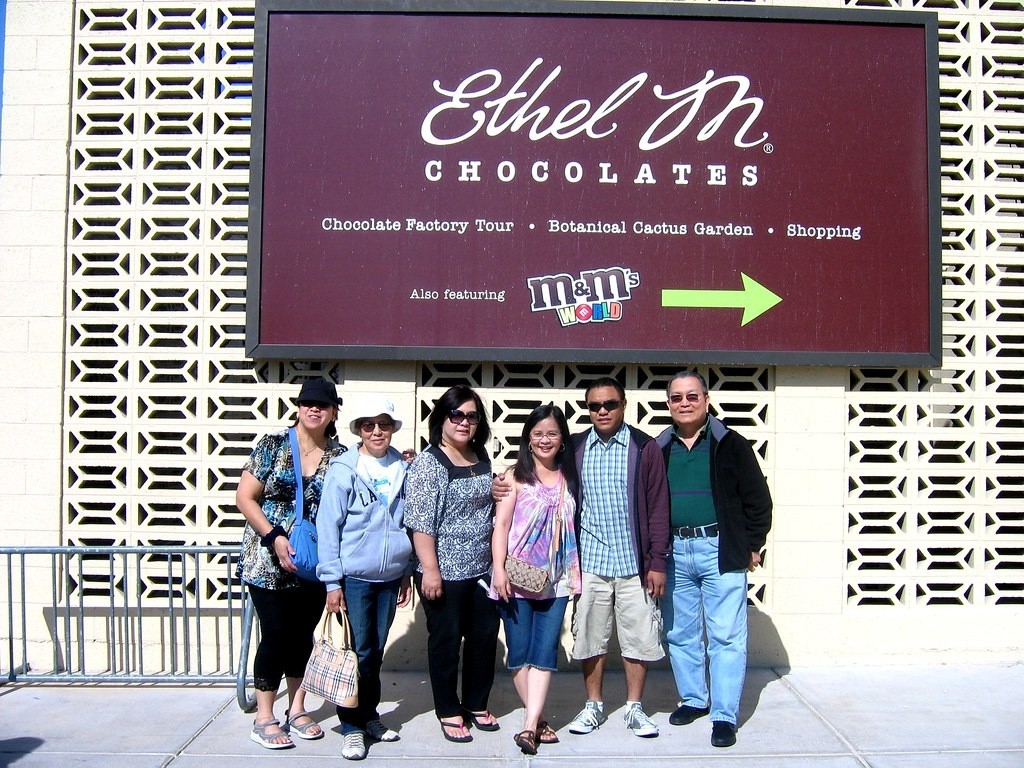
[403,386,500,744]
[492,405,582,754]
[317,400,412,761]
[236,378,416,749]
[657,370,772,747]
[492,378,671,737]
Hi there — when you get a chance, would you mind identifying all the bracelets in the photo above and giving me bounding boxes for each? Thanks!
[261,525,289,548]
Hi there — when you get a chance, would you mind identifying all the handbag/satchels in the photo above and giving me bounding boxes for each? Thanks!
[488,555,551,595]
[289,518,321,582]
[300,604,359,708]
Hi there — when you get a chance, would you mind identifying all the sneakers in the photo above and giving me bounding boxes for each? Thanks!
[342,733,367,760]
[366,720,401,741]
[624,703,659,737]
[569,701,609,734]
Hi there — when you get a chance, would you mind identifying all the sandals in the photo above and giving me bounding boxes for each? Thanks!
[285,709,323,740]
[252,719,294,749]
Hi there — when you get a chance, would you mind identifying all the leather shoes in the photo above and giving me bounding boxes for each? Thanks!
[669,705,710,725]
[711,721,736,747]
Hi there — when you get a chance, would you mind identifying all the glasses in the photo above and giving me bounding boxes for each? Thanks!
[585,400,622,412]
[671,394,706,403]
[530,430,561,440]
[448,410,481,425]
[360,421,392,432]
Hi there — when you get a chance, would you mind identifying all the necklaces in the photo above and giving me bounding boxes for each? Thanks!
[443,441,476,477]
[296,427,326,456]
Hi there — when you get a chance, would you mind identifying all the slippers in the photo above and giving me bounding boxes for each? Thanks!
[514,721,558,755]
[467,709,500,731]
[440,720,473,743]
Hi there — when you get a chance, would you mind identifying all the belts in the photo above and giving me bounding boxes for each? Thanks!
[673,523,720,538]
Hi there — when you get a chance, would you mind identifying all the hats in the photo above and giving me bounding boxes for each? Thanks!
[295,380,338,408]
[349,395,402,436]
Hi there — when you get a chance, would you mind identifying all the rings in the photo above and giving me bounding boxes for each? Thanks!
[496,592,499,594]
[279,562,282,565]
